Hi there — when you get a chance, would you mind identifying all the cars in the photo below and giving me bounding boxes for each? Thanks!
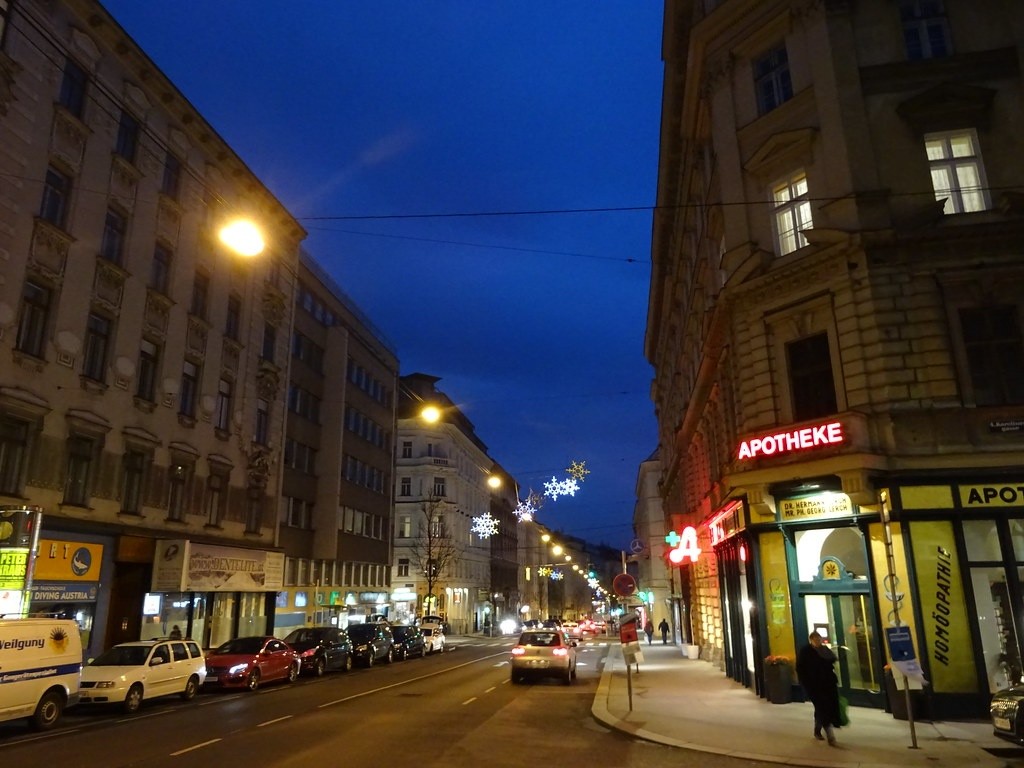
[421,616,443,628]
[198,637,301,691]
[990,684,1024,747]
[344,622,394,668]
[509,628,577,685]
[499,619,562,634]
[283,626,353,677]
[562,622,583,641]
[418,627,446,655]
[389,625,428,661]
[579,621,596,633]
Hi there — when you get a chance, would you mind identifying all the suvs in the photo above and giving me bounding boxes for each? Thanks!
[77,637,207,713]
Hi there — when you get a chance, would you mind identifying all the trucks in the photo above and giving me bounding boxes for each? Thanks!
[0,612,81,731]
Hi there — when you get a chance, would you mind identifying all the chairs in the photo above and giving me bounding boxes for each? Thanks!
[550,636,559,645]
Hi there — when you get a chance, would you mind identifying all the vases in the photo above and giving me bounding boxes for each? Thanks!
[765,665,792,704]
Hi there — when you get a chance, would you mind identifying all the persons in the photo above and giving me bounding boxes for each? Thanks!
[170,625,182,641]
[796,631,846,746]
[548,615,563,627]
[644,618,654,646]
[658,619,670,644]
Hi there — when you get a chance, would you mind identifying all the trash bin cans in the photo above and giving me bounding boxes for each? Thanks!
[763,658,793,704]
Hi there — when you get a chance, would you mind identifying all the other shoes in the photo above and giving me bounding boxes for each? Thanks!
[827,738,837,747]
[814,733,824,740]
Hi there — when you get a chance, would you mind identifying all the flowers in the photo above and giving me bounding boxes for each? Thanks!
[764,655,790,666]
